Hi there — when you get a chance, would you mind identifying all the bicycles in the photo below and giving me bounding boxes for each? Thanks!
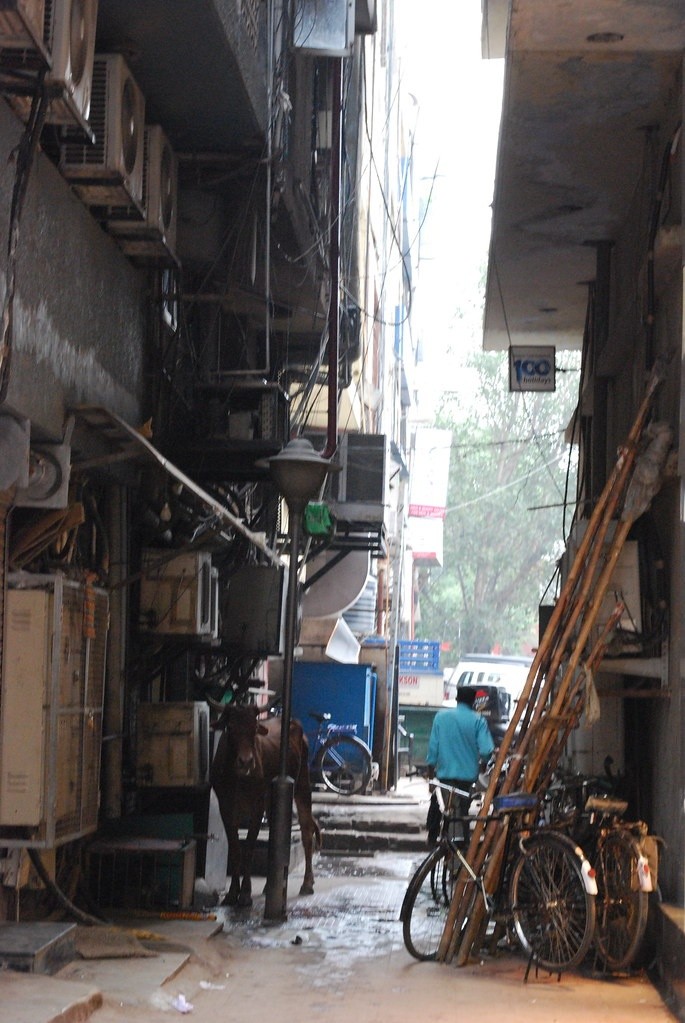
[307,710,374,798]
[398,744,669,984]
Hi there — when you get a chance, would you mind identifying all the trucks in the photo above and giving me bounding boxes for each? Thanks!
[444,652,550,734]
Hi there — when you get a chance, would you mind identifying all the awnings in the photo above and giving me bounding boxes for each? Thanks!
[80,402,285,568]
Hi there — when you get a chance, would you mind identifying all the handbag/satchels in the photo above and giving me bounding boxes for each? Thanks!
[426,788,441,845]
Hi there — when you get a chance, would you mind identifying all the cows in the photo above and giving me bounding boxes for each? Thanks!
[199,687,321,906]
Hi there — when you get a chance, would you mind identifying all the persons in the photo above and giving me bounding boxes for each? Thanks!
[426,687,493,878]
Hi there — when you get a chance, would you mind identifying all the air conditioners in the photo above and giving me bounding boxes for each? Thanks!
[82,836,198,916]
[137,547,222,639]
[0,1,182,263]
[134,698,212,788]
